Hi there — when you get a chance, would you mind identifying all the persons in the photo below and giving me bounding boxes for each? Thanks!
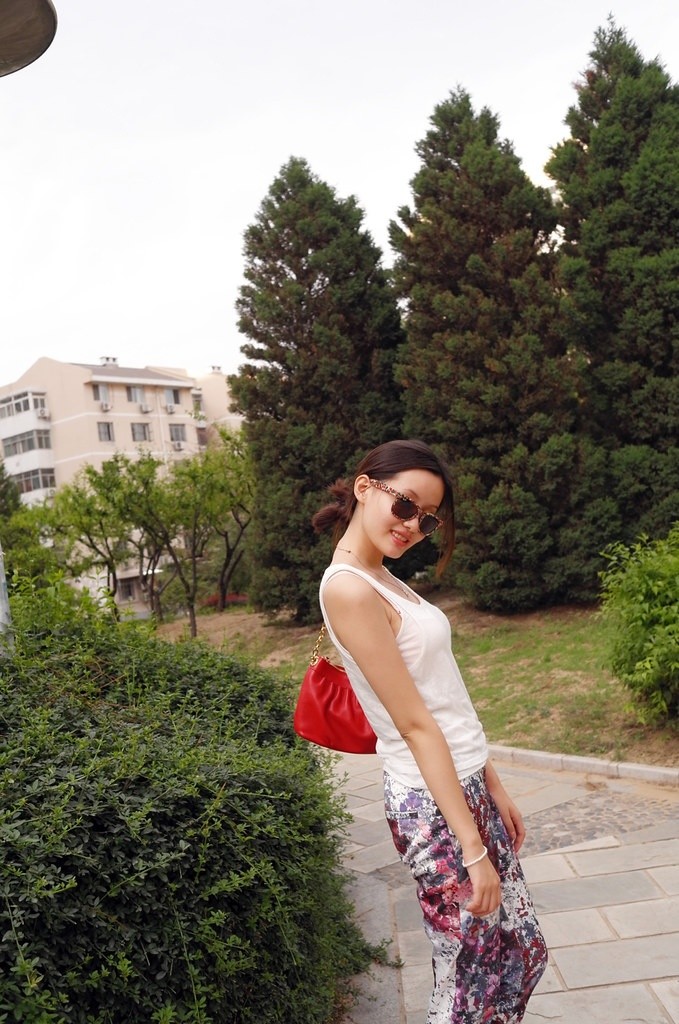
[318,439,550,1024]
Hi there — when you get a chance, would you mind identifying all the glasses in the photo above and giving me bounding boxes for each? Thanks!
[370,479,443,536]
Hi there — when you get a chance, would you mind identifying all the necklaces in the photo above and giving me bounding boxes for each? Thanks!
[336,545,409,598]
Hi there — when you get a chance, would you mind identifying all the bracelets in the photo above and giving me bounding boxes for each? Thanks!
[462,845,488,869]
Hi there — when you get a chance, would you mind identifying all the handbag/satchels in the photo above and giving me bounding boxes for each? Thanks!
[293,622,377,754]
